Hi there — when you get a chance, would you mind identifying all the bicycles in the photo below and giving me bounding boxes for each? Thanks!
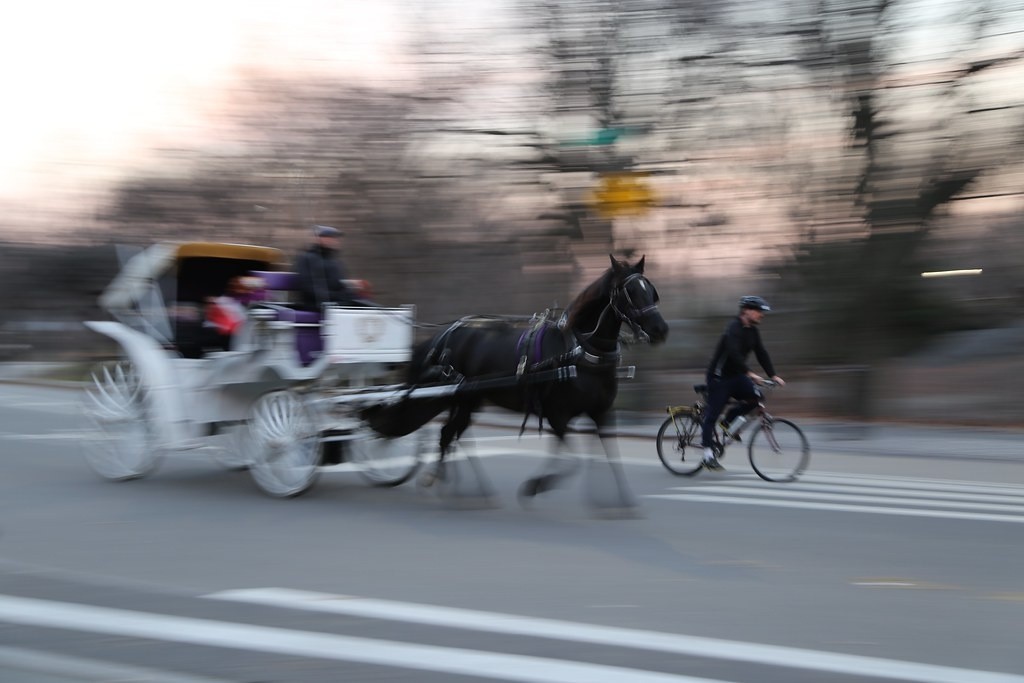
[654,374,811,485]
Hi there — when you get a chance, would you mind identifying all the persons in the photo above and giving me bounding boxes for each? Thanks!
[288,225,351,310]
[701,294,785,471]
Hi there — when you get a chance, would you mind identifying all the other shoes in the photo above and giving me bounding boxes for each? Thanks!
[700,453,725,472]
[719,419,743,442]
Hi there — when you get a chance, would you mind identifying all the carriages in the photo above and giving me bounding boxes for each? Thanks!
[69,238,672,521]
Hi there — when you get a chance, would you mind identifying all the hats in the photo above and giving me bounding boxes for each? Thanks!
[314,226,345,238]
[739,295,771,311]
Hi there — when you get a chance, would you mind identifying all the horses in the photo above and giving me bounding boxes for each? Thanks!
[372,253,669,521]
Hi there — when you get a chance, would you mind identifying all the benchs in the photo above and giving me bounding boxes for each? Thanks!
[248,269,325,365]
